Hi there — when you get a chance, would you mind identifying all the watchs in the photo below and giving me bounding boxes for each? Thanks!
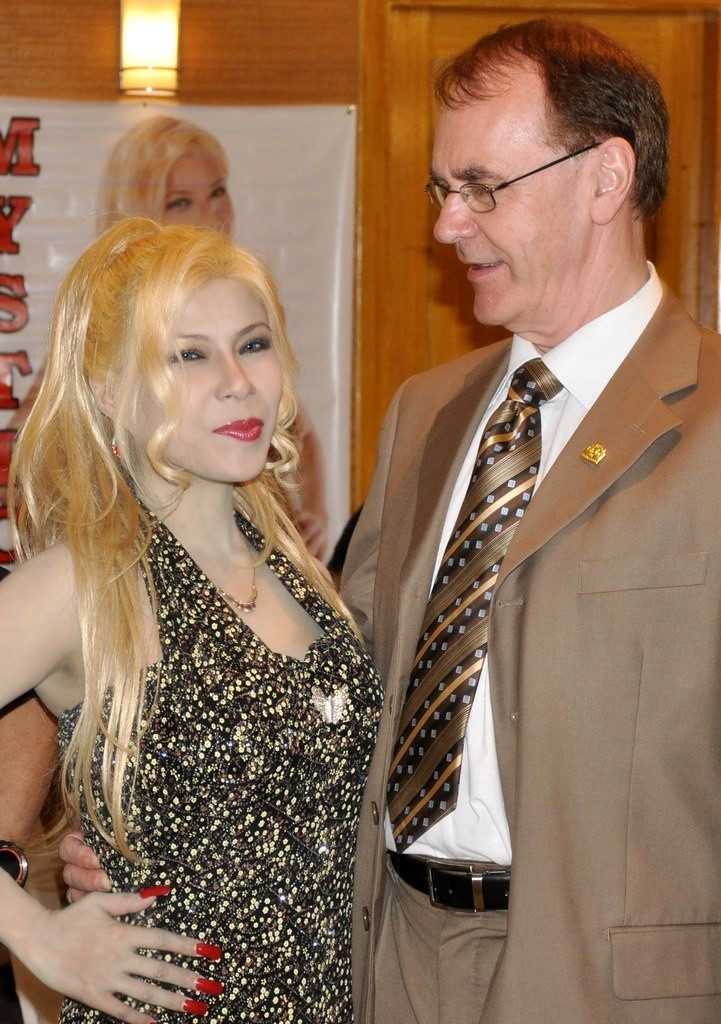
[0,839,28,889]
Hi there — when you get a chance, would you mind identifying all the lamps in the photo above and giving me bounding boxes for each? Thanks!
[118,0,181,98]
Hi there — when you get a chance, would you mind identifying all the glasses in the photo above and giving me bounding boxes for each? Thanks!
[423,141,601,213]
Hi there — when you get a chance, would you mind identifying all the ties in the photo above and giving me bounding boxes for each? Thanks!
[387,356,563,853]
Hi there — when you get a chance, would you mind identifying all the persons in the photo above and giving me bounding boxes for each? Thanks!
[59,18,717,1021]
[96,113,325,563]
[0,220,383,1024]
[0,561,62,1024]
